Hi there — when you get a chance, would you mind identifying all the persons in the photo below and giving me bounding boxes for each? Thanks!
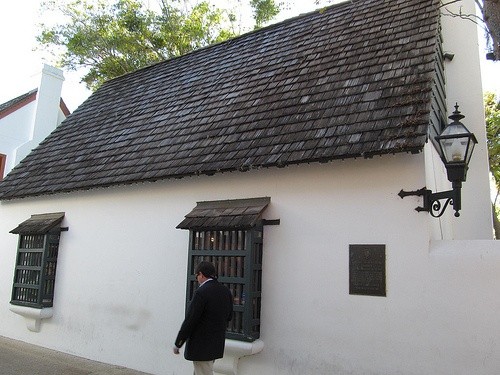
[172,261,233,375]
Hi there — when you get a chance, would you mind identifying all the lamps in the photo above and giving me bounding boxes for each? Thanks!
[397,102,478,218]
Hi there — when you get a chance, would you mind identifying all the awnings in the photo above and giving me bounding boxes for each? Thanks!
[8,212,65,235]
[175,197,271,230]
